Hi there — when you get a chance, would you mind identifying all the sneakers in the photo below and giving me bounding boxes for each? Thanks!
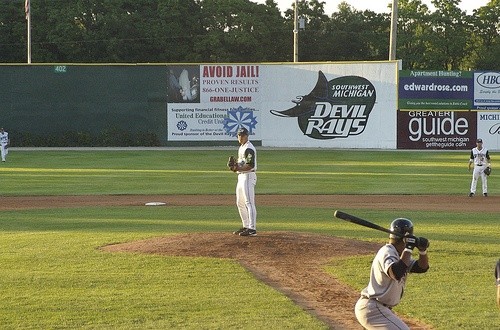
[239,229,257,237]
[233,228,247,235]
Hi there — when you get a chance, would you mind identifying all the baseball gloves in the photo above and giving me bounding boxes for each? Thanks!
[483,167,492,176]
[226,155,239,173]
[5,144,9,149]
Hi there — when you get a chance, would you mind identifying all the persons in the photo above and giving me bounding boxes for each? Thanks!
[354,218,430,330]
[0,128,11,162]
[468,138,491,197]
[229,127,258,236]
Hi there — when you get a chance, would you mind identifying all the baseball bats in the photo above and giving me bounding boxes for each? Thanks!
[333,208,430,248]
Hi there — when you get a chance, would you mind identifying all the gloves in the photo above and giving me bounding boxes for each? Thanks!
[415,236,430,254]
[403,233,418,253]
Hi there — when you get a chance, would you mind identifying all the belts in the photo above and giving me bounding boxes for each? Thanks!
[362,295,392,310]
[251,170,254,172]
[477,164,483,166]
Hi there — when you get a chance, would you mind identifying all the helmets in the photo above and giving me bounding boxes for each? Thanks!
[389,217,416,239]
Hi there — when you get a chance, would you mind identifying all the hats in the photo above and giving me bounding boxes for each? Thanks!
[237,127,249,136]
[477,139,482,143]
[1,127,4,130]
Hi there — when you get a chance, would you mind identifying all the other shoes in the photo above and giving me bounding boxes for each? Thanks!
[483,193,488,197]
[2,159,6,162]
[470,193,474,197]
